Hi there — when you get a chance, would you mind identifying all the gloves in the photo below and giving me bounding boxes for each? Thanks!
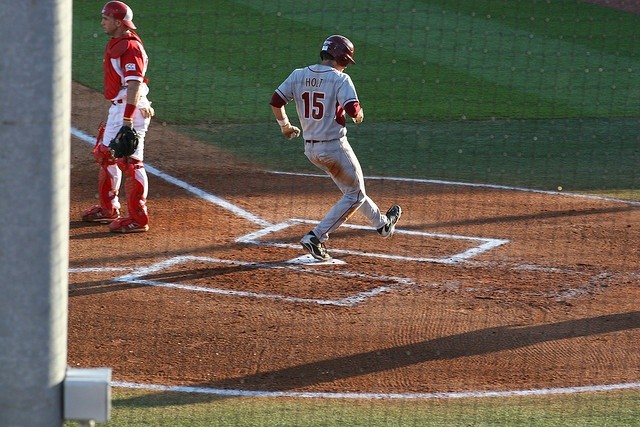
[352,108,364,124]
[140,107,155,119]
[276,116,301,140]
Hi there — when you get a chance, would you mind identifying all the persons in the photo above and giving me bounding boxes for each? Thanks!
[268,33,402,261]
[78,1,155,232]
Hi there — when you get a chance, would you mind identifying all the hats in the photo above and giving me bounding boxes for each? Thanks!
[101,1,138,30]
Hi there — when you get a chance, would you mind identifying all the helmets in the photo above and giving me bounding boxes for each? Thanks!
[319,35,356,64]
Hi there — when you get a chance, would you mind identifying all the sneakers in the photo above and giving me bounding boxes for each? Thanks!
[377,204,401,240]
[108,216,149,233]
[300,230,331,260]
[82,205,121,222]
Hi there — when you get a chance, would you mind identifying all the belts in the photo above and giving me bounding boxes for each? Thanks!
[306,140,325,143]
[111,99,122,105]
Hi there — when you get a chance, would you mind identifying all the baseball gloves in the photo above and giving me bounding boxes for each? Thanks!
[109,126,138,157]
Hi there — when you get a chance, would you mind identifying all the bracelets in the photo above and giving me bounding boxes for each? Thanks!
[123,102,137,121]
[275,116,289,126]
